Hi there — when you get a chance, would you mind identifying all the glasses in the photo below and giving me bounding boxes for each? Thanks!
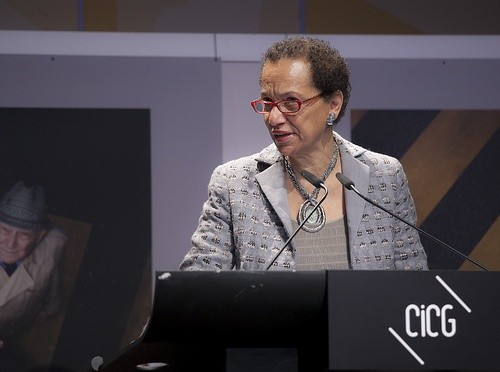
[250,94,327,115]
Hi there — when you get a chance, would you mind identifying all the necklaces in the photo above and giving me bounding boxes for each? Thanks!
[285,143,340,233]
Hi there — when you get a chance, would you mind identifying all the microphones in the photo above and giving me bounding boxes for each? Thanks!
[266,170,329,271]
[336,172,489,271]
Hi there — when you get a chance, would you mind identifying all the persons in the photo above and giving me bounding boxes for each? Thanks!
[179,37,429,271]
[0,183,67,344]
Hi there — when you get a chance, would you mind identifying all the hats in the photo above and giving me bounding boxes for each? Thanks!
[0,183,55,231]
